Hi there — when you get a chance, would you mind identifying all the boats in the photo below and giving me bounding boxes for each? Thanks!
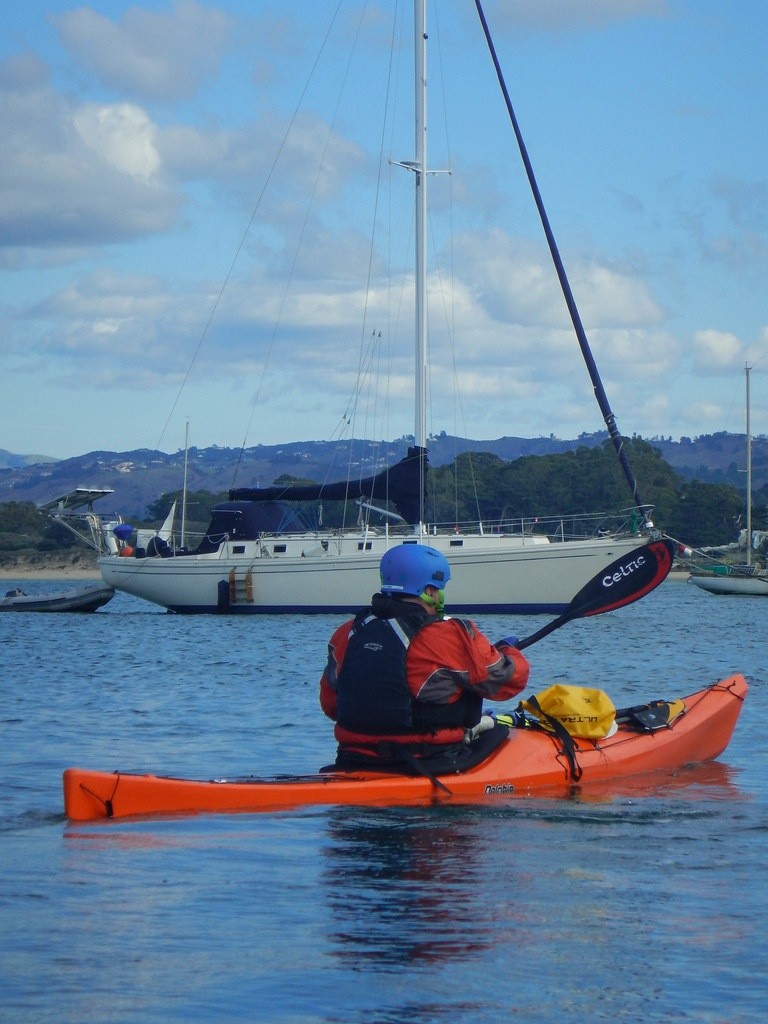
[61,667,757,824]
[0,581,120,615]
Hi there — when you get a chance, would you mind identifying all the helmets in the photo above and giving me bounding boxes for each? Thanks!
[375,544,453,599]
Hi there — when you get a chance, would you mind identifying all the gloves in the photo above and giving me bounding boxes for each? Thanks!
[493,634,518,651]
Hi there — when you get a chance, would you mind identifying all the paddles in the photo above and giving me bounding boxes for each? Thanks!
[516,538,674,652]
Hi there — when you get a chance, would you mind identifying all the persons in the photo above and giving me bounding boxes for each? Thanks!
[317,543,531,773]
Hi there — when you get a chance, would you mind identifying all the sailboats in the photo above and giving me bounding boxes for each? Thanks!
[688,363,766,596]
[96,1,672,618]
